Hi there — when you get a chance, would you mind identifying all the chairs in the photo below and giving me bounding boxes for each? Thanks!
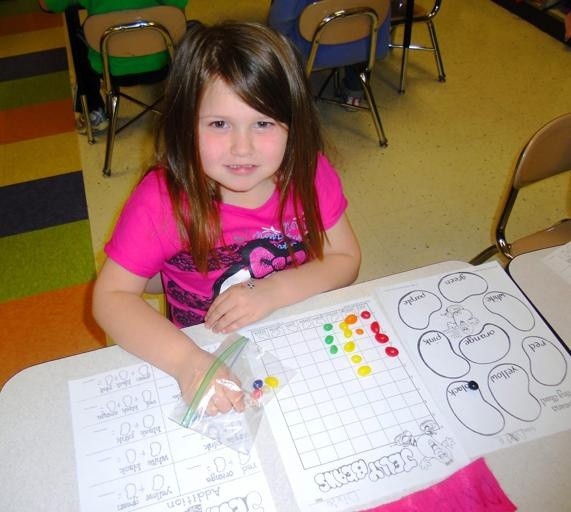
[389,1,444,95]
[299,1,390,148]
[83,6,187,176]
[469,112,571,265]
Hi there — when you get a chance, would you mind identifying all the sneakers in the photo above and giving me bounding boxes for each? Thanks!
[74,108,109,133]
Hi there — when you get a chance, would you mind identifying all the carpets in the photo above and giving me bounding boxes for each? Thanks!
[0,0,107,391]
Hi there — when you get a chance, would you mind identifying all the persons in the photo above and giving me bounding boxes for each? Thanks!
[91,15,364,416]
[38,0,190,136]
[267,0,393,112]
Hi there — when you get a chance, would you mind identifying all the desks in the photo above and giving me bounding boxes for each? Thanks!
[506,241,571,354]
[0,261,571,511]
